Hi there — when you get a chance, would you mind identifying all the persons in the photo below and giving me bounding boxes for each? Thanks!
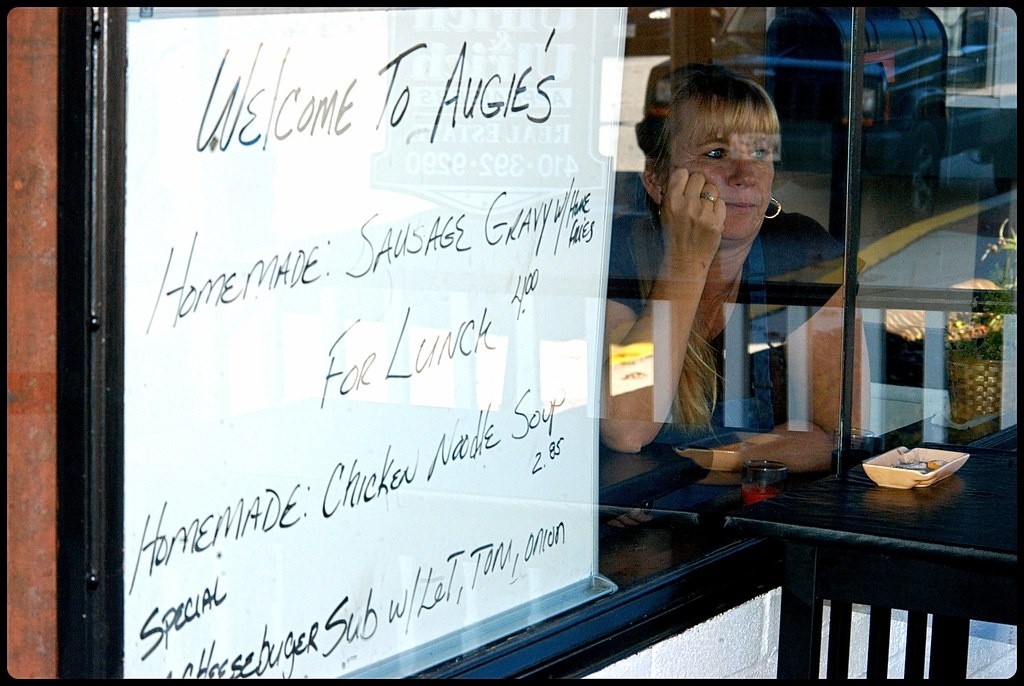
[595,62,872,485]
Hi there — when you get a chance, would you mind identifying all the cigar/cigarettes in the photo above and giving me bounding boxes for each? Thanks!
[898,461,942,470]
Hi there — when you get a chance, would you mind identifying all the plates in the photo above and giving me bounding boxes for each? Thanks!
[863,447,970,489]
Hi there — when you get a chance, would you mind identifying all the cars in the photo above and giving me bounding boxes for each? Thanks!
[637,4,1017,228]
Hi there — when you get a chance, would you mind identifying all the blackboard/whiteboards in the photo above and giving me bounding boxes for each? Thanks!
[89,6,628,680]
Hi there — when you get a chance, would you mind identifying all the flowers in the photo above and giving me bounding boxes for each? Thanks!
[945,266,1016,360]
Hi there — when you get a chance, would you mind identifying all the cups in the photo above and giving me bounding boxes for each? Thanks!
[831,426,874,473]
[741,460,787,506]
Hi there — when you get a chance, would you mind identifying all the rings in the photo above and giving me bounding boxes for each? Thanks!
[699,192,717,205]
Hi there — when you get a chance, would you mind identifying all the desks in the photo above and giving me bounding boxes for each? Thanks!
[598,420,1021,684]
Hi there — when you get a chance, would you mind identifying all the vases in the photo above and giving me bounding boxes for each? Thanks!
[947,358,1002,441]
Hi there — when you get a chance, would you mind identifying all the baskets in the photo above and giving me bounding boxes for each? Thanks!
[948,349,1002,442]
